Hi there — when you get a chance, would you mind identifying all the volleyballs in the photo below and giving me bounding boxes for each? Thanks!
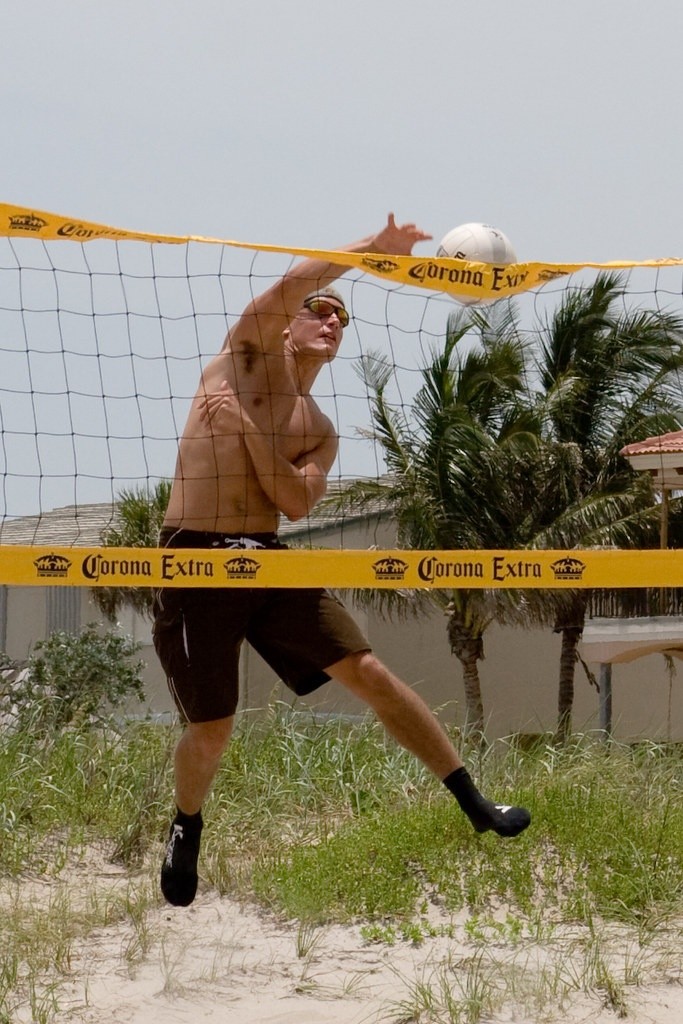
[435,222,518,310]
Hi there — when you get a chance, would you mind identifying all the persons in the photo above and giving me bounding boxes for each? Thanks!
[151,210,534,908]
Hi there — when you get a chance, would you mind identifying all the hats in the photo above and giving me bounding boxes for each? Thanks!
[303,286,344,307]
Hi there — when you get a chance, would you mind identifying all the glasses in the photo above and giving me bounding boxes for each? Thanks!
[302,301,349,328]
